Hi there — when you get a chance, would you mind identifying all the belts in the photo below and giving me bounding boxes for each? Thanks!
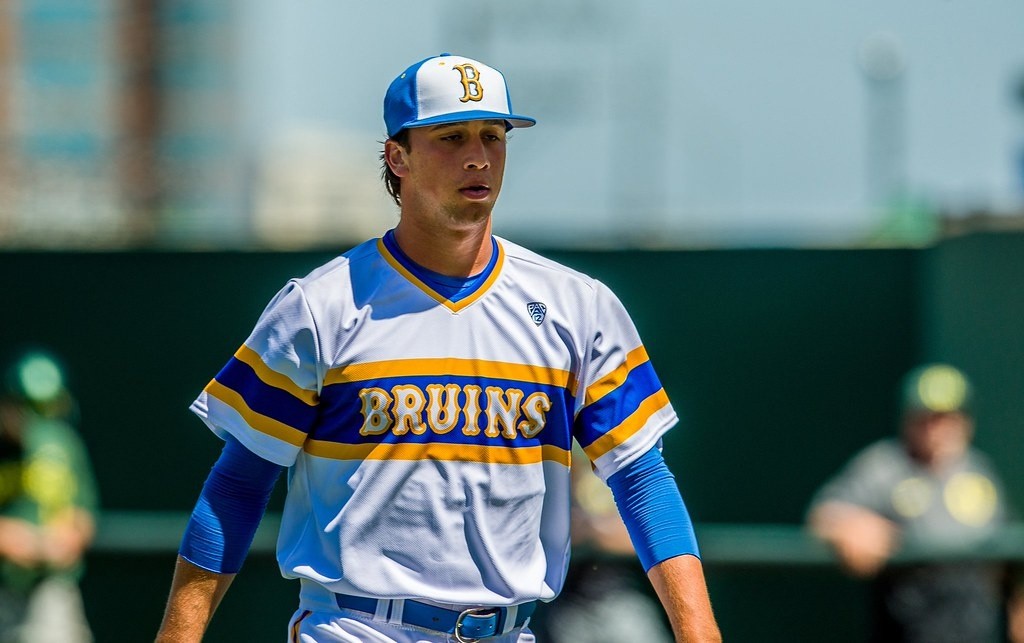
[335,591,537,643]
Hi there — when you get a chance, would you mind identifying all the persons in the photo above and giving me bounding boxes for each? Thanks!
[0,351,101,643]
[155,52,724,642]
[805,361,1024,643]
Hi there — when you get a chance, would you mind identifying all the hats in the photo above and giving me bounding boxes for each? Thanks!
[382,52,536,138]
[5,352,78,423]
[893,357,978,414]
